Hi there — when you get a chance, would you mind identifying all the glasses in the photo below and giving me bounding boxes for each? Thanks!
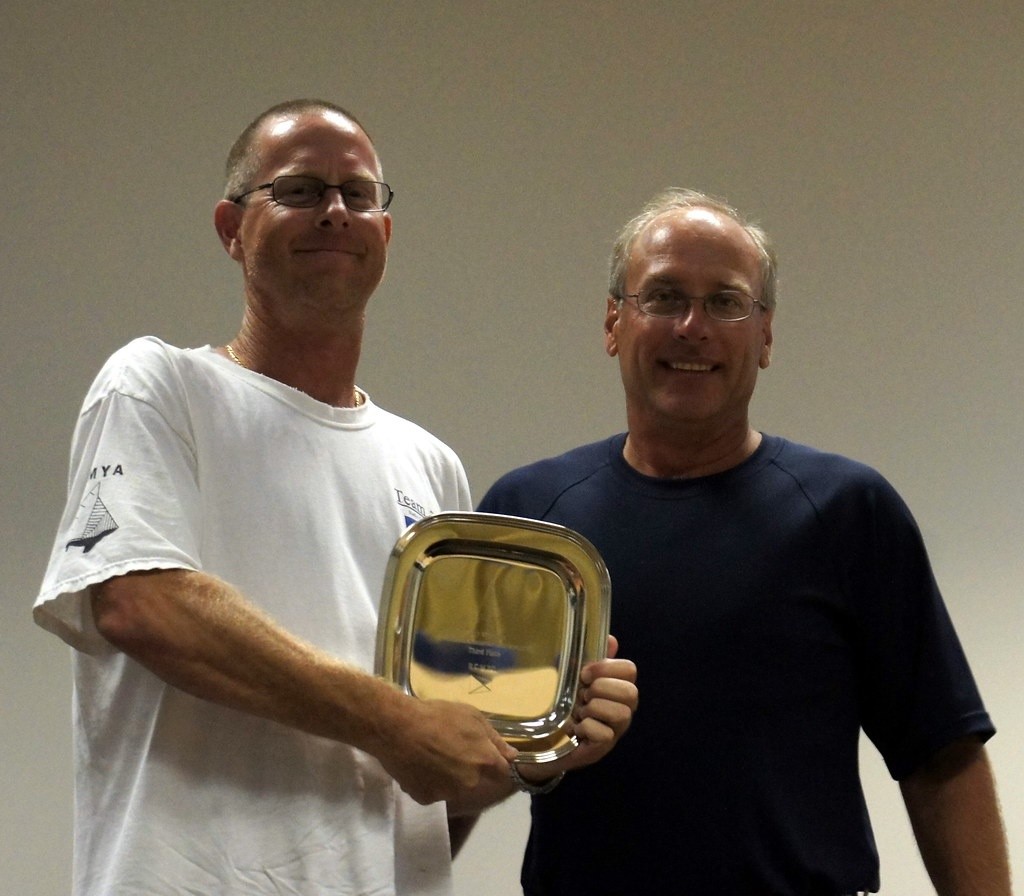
[231,172,398,209]
[609,278,770,325]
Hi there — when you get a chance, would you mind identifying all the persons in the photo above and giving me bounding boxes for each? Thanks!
[33,97,641,896]
[472,185,1012,896]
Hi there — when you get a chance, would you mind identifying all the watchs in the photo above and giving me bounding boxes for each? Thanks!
[506,726,569,795]
